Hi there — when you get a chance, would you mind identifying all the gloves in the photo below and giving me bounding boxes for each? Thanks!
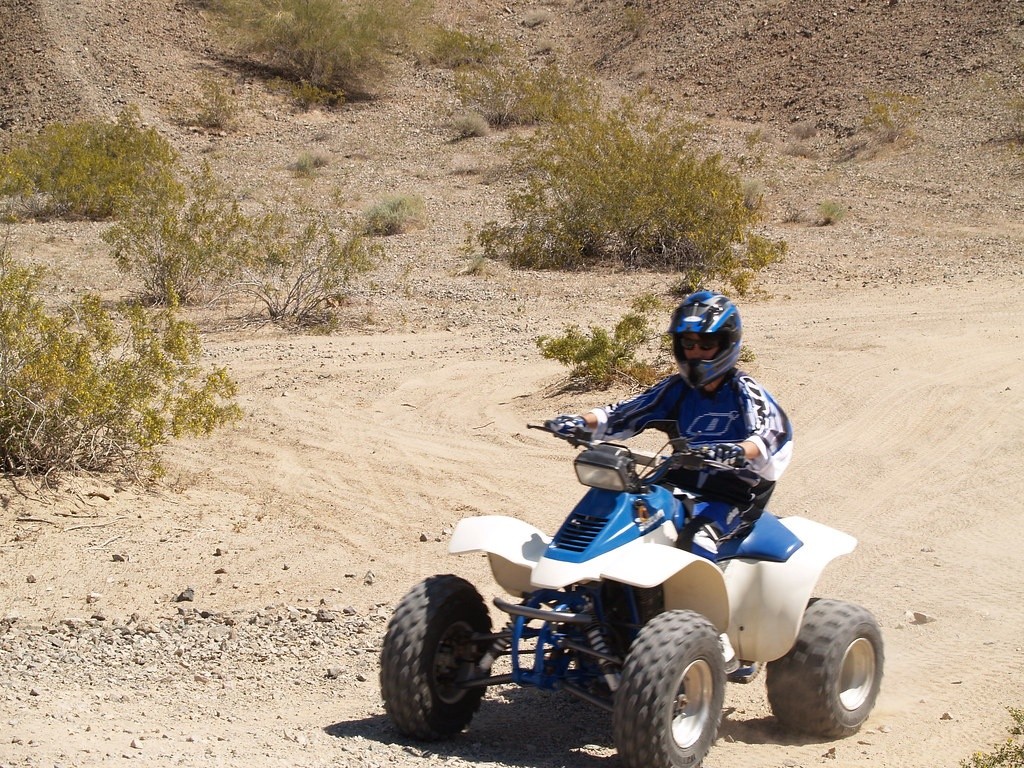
[544,414,584,442]
[699,440,754,470]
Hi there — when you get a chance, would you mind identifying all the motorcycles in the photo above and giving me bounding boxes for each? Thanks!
[378,418,887,768]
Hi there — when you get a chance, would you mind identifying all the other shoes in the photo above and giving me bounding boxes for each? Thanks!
[716,633,741,675]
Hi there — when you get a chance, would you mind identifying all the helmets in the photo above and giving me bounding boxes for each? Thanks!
[665,292,743,389]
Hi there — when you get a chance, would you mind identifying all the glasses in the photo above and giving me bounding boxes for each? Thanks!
[679,336,721,352]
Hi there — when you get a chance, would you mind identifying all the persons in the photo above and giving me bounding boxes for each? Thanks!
[544,292,795,676]
